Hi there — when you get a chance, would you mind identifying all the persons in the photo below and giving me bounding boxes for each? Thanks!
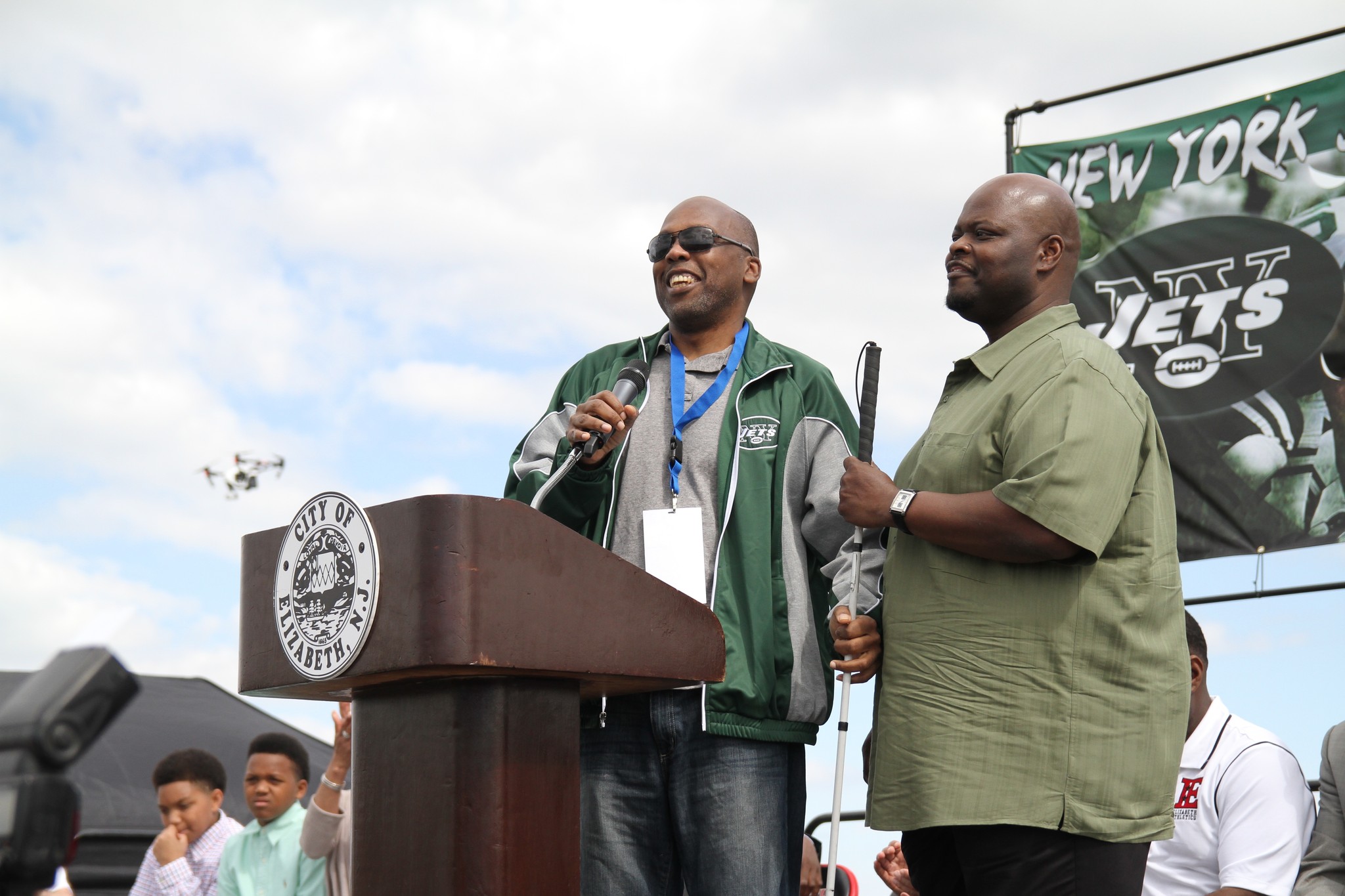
[128,749,245,896]
[837,172,1189,896]
[503,197,889,896]
[299,702,351,896]
[216,733,327,896]
[798,605,1345,896]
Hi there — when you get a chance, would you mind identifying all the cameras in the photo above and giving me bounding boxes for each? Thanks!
[0,645,144,893]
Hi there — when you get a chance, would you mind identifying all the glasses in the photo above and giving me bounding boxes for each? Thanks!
[646,228,754,263]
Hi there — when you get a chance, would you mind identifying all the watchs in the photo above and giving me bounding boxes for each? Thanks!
[890,489,922,536]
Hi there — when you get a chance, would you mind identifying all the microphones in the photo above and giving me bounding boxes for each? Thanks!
[582,360,650,458]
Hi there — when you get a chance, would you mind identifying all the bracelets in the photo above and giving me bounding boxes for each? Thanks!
[320,773,346,791]
